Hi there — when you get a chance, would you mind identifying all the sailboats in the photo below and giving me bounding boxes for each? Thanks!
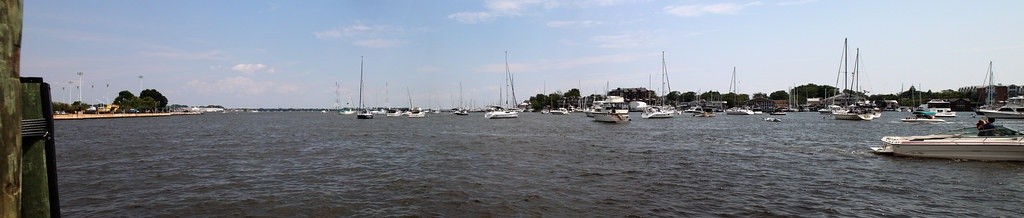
[337,56,439,118]
[452,75,534,115]
[485,51,518,118]
[642,51,676,118]
[830,37,882,120]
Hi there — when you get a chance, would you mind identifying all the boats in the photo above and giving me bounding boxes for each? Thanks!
[869,136,1024,161]
[901,114,945,124]
[725,107,762,115]
[541,81,630,122]
[913,99,956,117]
[682,106,715,117]
[975,103,1024,118]
